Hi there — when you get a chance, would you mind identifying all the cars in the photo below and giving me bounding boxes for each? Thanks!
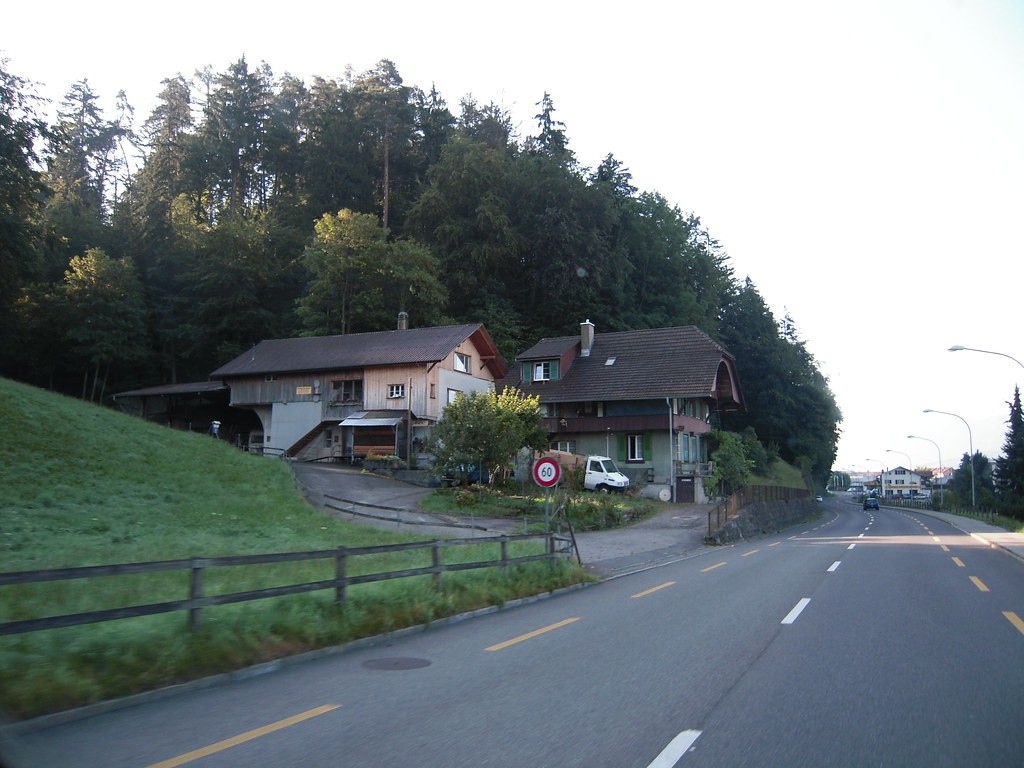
[863,498,880,510]
[903,494,911,499]
[870,489,882,495]
[816,496,823,504]
[431,459,490,489]
[892,494,904,499]
[847,488,858,492]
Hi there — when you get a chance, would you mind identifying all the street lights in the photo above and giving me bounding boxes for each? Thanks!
[947,344,1024,367]
[906,435,943,504]
[923,409,976,506]
[852,464,870,490]
[886,450,913,500]
[866,458,888,503]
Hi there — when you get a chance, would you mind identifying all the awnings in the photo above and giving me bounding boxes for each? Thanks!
[339,417,403,426]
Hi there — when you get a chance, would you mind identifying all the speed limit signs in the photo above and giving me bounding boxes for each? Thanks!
[534,456,561,488]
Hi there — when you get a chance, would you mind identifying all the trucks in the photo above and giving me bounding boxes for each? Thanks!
[531,450,630,496]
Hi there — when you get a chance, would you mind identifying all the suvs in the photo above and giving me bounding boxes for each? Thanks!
[914,493,930,500]
